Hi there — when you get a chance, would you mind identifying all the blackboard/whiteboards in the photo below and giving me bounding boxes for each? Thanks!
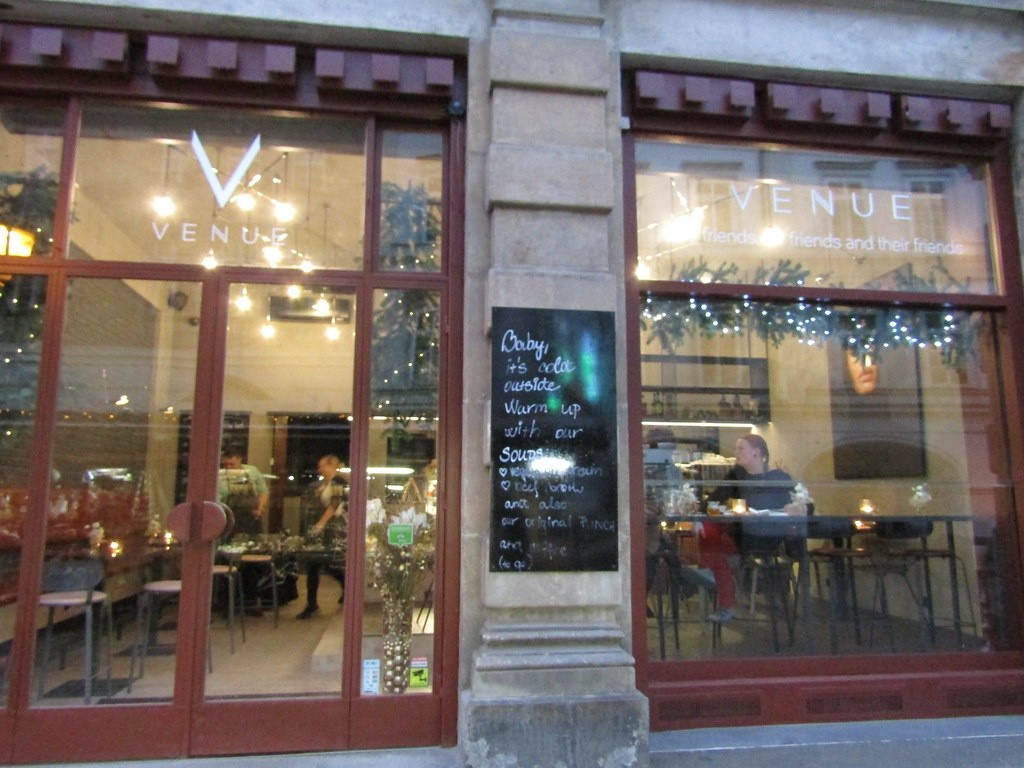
[489,306,620,575]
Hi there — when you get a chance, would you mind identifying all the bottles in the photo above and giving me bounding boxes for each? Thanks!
[718,394,744,420]
[80,520,103,549]
[148,513,161,537]
[793,483,816,516]
[641,391,663,417]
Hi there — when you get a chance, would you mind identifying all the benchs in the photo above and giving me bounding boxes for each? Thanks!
[0,487,166,643]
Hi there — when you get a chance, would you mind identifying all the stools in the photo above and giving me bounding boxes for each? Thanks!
[40,551,113,702]
[235,553,279,628]
[646,519,860,654]
[867,515,935,651]
[210,563,247,653]
[128,549,212,690]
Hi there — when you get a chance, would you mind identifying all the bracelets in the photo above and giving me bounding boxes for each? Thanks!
[257,506,266,514]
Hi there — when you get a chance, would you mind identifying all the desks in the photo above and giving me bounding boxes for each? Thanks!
[645,513,972,654]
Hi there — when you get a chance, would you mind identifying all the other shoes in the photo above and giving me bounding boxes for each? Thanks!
[646,605,654,618]
[296,605,318,618]
[339,590,345,604]
[708,609,736,621]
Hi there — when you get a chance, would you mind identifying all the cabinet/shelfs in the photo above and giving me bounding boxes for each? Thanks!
[640,353,771,423]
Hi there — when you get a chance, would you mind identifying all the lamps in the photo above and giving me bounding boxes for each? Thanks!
[150,141,343,341]
[0,212,41,288]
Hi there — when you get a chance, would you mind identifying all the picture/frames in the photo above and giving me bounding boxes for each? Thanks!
[825,262,928,482]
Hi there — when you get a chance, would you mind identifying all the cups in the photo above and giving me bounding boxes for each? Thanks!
[665,493,679,516]
[708,501,719,514]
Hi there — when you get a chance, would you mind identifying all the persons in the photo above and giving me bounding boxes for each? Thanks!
[215,449,270,534]
[642,426,715,617]
[699,434,808,624]
[296,455,350,619]
